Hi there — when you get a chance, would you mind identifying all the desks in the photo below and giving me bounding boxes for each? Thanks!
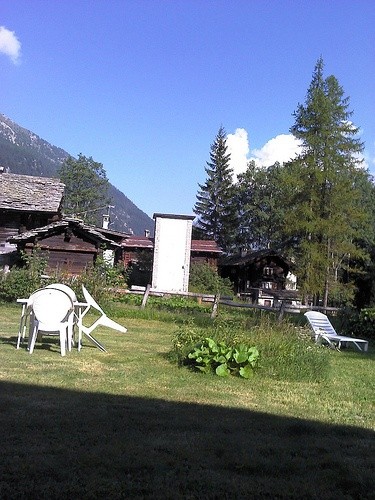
[16,298,91,354]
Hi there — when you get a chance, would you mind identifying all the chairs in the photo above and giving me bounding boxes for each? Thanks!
[76,283,128,354]
[26,288,74,358]
[304,310,369,352]
